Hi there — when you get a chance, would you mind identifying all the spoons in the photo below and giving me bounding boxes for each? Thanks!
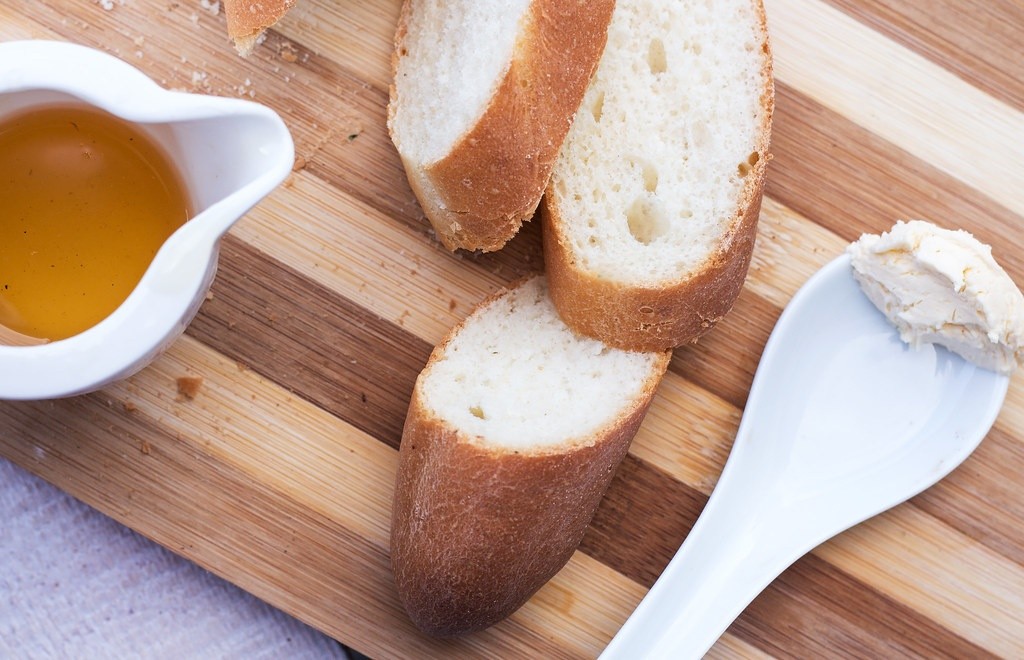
[593,249,1014,660]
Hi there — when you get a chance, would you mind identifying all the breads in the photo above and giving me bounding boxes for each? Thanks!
[389,270,676,637]
[542,1,777,350]
[384,1,618,254]
[223,0,299,59]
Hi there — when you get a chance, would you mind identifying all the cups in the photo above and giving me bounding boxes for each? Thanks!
[0,41,296,398]
